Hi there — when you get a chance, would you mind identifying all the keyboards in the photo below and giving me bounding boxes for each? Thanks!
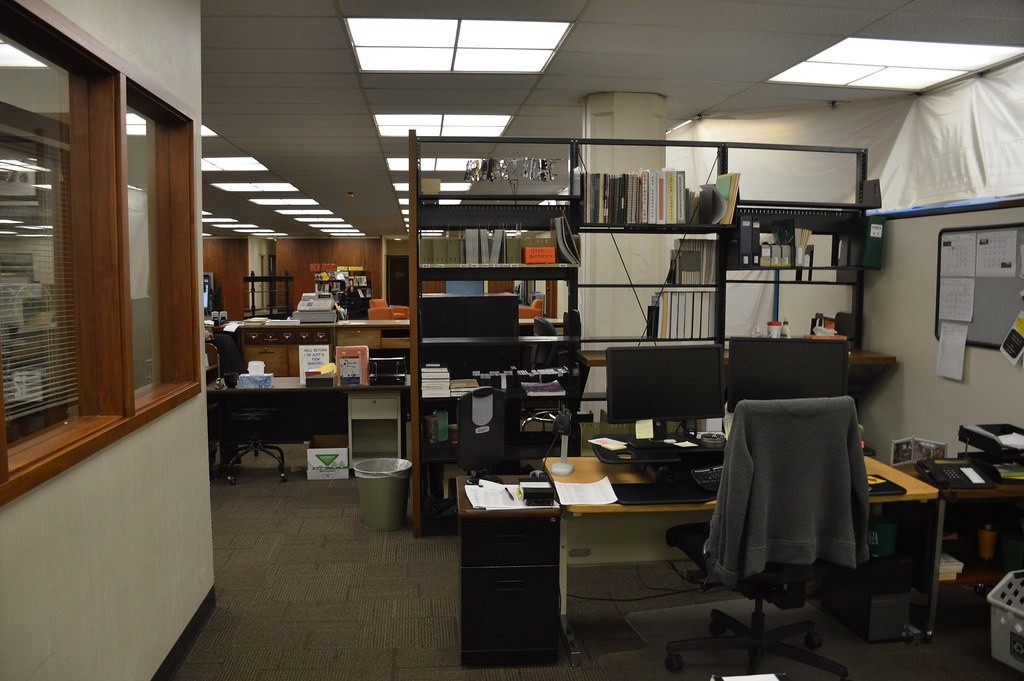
[692,463,723,490]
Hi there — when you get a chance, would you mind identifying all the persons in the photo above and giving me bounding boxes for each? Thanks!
[204,331,248,465]
[17,284,57,334]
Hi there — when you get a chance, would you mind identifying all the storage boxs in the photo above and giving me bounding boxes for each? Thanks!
[521,246,555,264]
[307,434,350,480]
[986,569,1024,675]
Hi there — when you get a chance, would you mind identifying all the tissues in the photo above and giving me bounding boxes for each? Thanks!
[238,359,274,388]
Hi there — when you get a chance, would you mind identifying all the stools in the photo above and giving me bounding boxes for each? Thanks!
[222,408,288,482]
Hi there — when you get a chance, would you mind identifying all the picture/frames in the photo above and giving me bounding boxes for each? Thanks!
[891,436,948,467]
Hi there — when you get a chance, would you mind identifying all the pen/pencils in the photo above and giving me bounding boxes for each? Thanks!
[505,488,515,501]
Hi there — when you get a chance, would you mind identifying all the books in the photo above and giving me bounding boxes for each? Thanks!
[520,379,565,396]
[420,364,479,397]
[647,291,715,340]
[669,238,715,286]
[581,169,741,224]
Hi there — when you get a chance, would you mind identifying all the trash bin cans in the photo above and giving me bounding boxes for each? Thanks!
[353,458,412,532]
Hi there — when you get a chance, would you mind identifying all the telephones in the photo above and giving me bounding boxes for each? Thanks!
[915,459,996,489]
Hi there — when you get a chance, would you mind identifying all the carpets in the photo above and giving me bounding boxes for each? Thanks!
[600,597,918,681]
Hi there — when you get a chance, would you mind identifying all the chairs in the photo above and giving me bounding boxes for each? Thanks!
[205,342,220,379]
[522,316,561,434]
[435,387,507,521]
[368,357,408,375]
[665,394,869,681]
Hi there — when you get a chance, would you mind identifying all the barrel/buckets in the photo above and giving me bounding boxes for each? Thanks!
[1001,534,1024,573]
[978,528,997,559]
[868,517,896,557]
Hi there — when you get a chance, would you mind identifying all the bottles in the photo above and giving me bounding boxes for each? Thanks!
[781,321,791,338]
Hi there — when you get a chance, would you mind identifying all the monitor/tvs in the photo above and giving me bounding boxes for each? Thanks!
[727,337,849,414]
[203,275,210,308]
[605,345,725,447]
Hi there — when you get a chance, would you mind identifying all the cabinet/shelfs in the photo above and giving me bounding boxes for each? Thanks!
[241,319,410,377]
[454,474,562,668]
[419,292,519,431]
[891,459,1024,645]
[314,271,371,310]
[408,130,869,539]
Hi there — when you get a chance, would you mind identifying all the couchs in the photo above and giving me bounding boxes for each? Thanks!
[368,299,409,319]
[519,299,543,318]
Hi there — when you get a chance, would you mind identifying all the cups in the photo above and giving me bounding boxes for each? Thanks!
[449,424,457,443]
[425,415,439,444]
[767,321,781,338]
[247,361,265,374]
[433,410,448,441]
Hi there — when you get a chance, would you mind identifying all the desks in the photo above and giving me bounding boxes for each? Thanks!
[576,349,896,414]
[206,374,410,469]
[518,318,564,327]
[541,455,938,668]
[205,321,240,354]
